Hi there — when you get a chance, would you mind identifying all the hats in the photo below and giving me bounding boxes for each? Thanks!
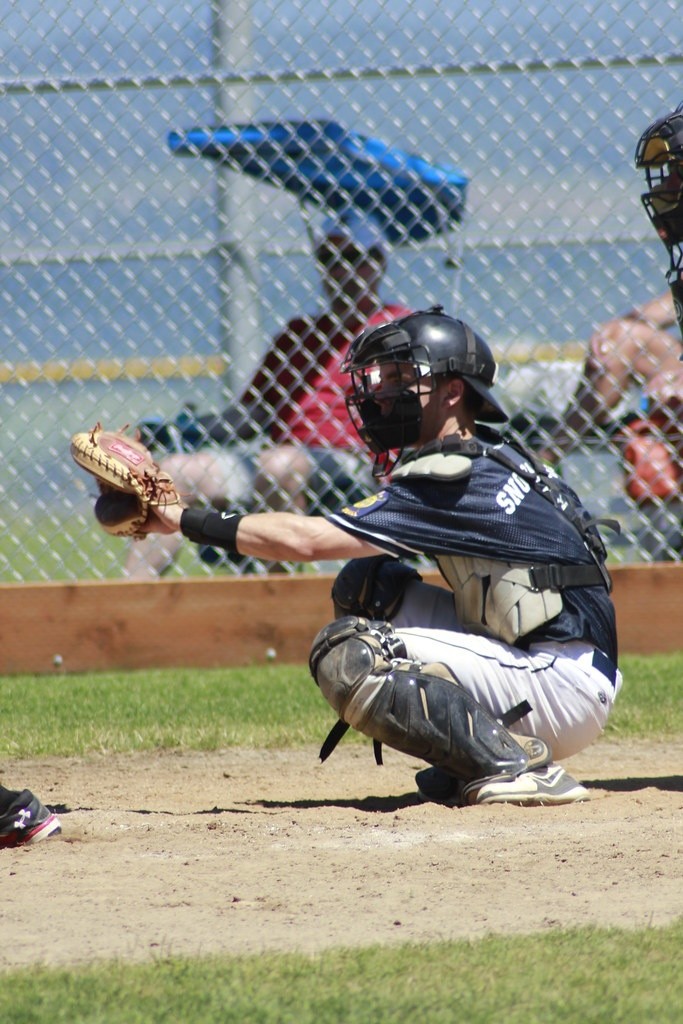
[315,207,390,261]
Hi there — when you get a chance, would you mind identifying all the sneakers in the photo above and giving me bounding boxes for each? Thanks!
[415,763,592,805]
[0,789,62,849]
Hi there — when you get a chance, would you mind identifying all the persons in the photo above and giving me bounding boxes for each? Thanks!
[78,303,625,807]
[515,105,683,569]
[101,220,416,573]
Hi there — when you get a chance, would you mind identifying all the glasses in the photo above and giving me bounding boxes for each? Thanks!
[316,249,382,267]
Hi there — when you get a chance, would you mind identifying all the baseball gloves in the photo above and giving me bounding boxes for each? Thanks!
[69,420,193,542]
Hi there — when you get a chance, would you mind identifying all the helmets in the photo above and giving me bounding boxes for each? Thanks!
[377,304,509,424]
[634,101,683,245]
